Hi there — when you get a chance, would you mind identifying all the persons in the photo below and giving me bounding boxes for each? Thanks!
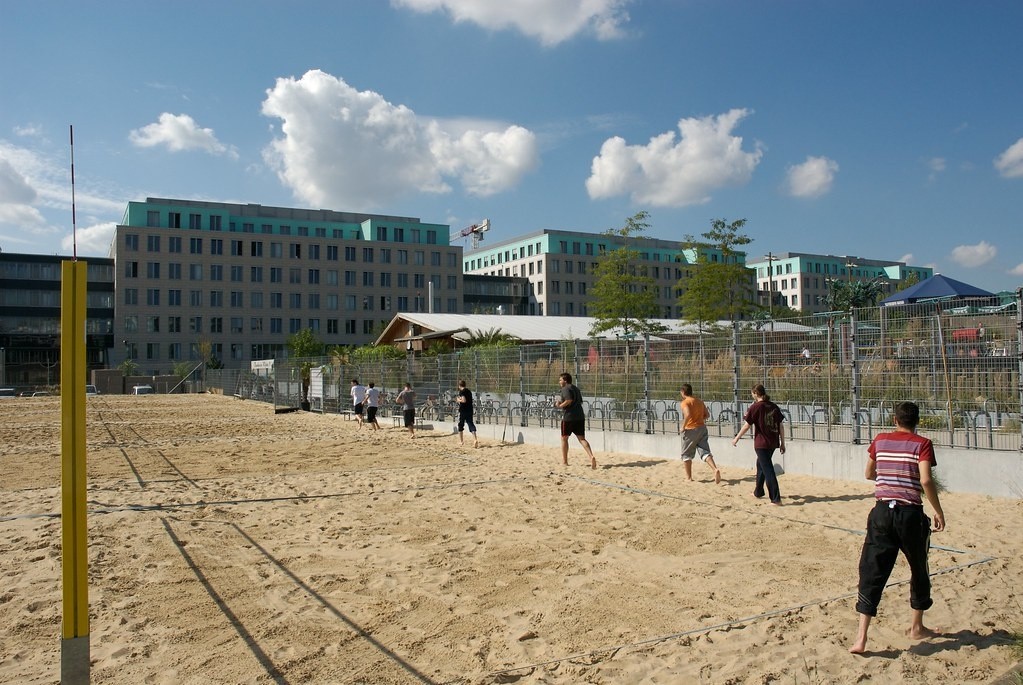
[396,383,416,439]
[800,347,811,365]
[733,384,786,504]
[960,322,964,329]
[977,322,985,356]
[362,382,380,431]
[456,380,478,448]
[556,373,596,469]
[351,380,367,427]
[680,384,721,484]
[850,401,946,654]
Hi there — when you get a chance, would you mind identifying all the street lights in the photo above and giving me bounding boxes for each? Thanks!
[764,252,779,319]
[845,257,858,286]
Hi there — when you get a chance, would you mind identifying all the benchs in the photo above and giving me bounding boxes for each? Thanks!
[343,410,365,423]
[391,416,425,431]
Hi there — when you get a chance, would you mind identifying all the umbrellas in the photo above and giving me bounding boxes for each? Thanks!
[944,290,1018,315]
[879,274,1000,433]
[816,317,881,355]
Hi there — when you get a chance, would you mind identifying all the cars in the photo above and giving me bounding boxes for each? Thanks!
[0,388,16,398]
[20,391,33,397]
[86,384,100,396]
[132,385,154,395]
[32,392,50,397]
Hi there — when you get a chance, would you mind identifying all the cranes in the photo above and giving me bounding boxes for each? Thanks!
[449,218,491,250]
